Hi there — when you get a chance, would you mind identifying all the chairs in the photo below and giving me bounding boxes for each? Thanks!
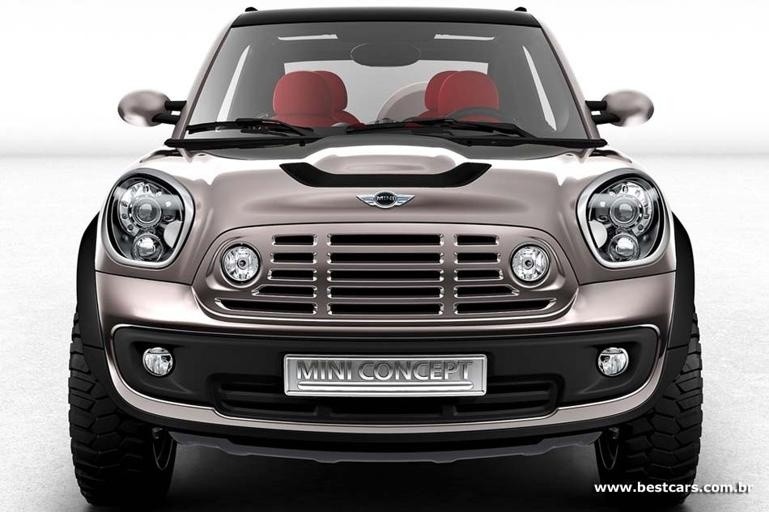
[268,71,500,133]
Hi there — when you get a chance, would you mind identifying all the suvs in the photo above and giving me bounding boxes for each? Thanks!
[63,4,707,509]
